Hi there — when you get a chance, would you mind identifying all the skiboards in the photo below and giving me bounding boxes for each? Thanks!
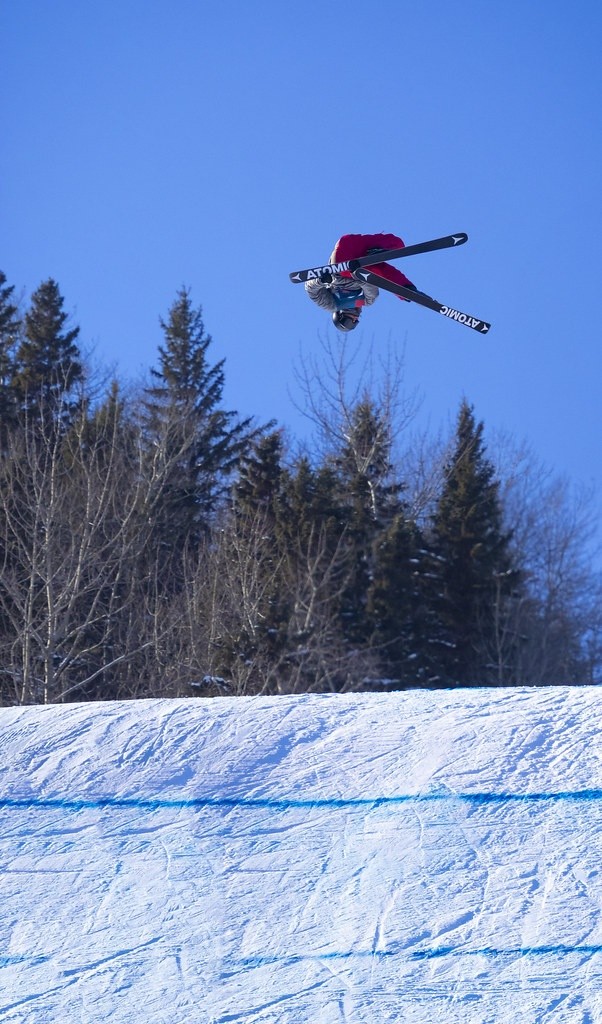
[289,232,491,336]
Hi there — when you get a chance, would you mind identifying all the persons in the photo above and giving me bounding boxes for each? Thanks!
[305,233,433,332]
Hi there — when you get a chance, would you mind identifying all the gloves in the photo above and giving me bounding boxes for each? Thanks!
[319,271,332,284]
[348,260,360,270]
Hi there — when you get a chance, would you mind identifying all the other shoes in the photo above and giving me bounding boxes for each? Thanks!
[404,284,433,301]
[366,248,390,256]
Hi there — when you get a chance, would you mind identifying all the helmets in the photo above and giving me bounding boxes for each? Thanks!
[332,307,362,332]
